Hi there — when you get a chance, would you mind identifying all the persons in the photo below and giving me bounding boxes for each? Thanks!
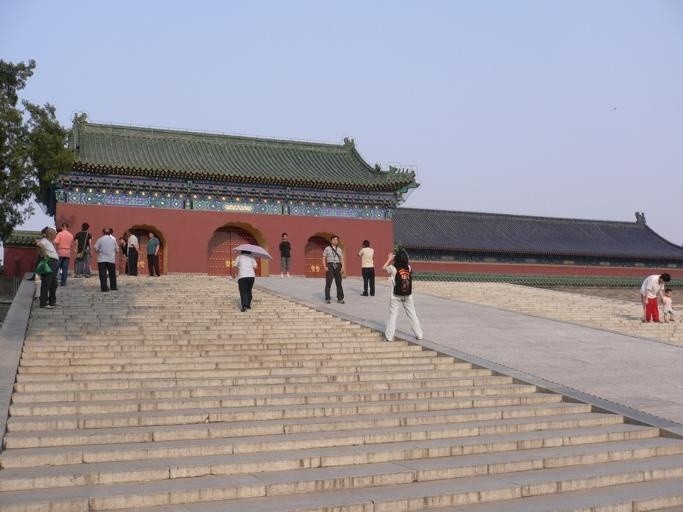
[322,236,346,305]
[233,251,257,312]
[147,232,160,277]
[662,288,675,322]
[358,240,375,296]
[382,247,424,342]
[640,273,671,323]
[27,221,140,309]
[279,233,291,278]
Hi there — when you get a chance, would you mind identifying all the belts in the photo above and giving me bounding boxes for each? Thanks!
[328,262,341,265]
[42,256,59,261]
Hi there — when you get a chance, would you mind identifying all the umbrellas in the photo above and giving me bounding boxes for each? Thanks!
[233,244,274,261]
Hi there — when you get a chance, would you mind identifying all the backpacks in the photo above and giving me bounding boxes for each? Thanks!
[393,264,412,296]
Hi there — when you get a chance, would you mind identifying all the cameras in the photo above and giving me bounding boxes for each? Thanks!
[332,263,338,270]
[393,255,396,261]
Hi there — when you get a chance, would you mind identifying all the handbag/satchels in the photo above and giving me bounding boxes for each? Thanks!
[75,250,84,260]
[35,257,53,274]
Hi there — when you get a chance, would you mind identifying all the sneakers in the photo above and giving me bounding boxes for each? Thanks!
[50,303,61,306]
[241,303,248,312]
[325,300,330,304]
[40,305,54,309]
[337,300,345,304]
